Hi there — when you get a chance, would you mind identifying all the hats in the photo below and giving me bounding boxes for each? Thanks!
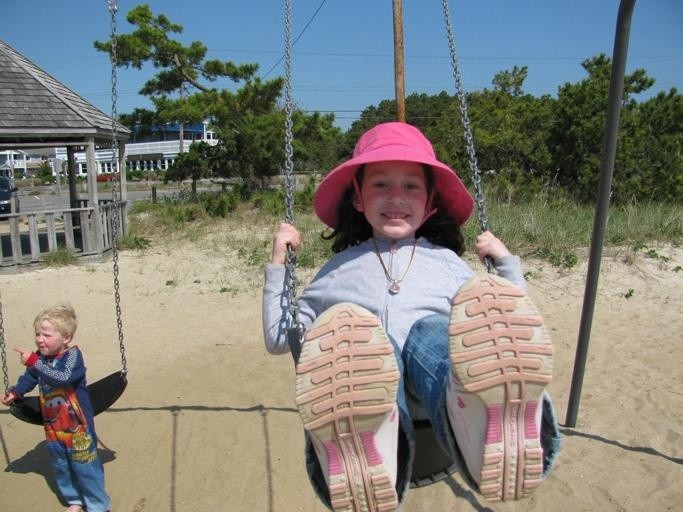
[314,122,476,230]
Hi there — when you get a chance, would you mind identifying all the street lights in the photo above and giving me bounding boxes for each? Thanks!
[203,121,207,143]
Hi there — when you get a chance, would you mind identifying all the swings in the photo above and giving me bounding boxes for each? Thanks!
[0,1,129,422]
[286,0,495,493]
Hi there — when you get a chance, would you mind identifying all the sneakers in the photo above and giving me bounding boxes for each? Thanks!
[447,271,554,503]
[294,301,402,512]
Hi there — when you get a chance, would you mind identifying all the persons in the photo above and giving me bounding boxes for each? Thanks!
[1,303,111,512]
[262,123,561,512]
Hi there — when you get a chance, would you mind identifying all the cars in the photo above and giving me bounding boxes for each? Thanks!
[0,175,21,220]
[97,175,115,181]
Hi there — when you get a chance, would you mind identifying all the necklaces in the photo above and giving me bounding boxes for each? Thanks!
[373,238,415,294]
